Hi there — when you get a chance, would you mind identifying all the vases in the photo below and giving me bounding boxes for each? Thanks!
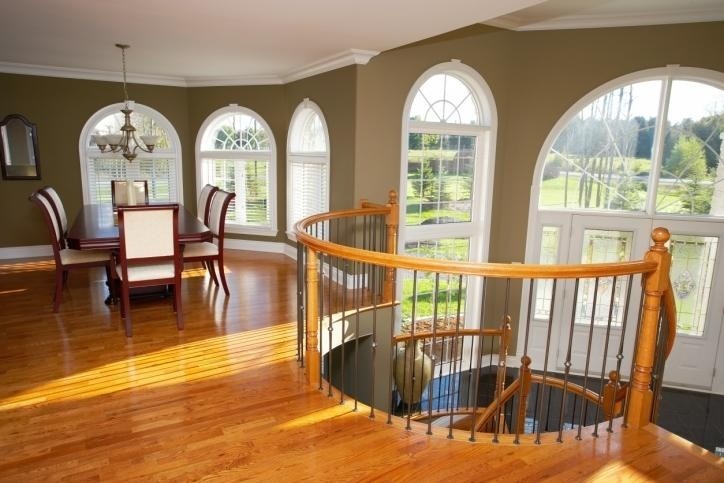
[392,340,432,405]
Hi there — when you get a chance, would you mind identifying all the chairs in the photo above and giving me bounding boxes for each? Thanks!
[110,203,184,337]
[181,189,236,296]
[196,184,219,270]
[111,180,149,207]
[38,185,71,248]
[28,190,115,313]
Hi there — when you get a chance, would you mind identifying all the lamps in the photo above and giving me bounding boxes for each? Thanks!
[94,43,159,163]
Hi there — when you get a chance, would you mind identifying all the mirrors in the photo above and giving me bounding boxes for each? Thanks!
[0,114,41,180]
